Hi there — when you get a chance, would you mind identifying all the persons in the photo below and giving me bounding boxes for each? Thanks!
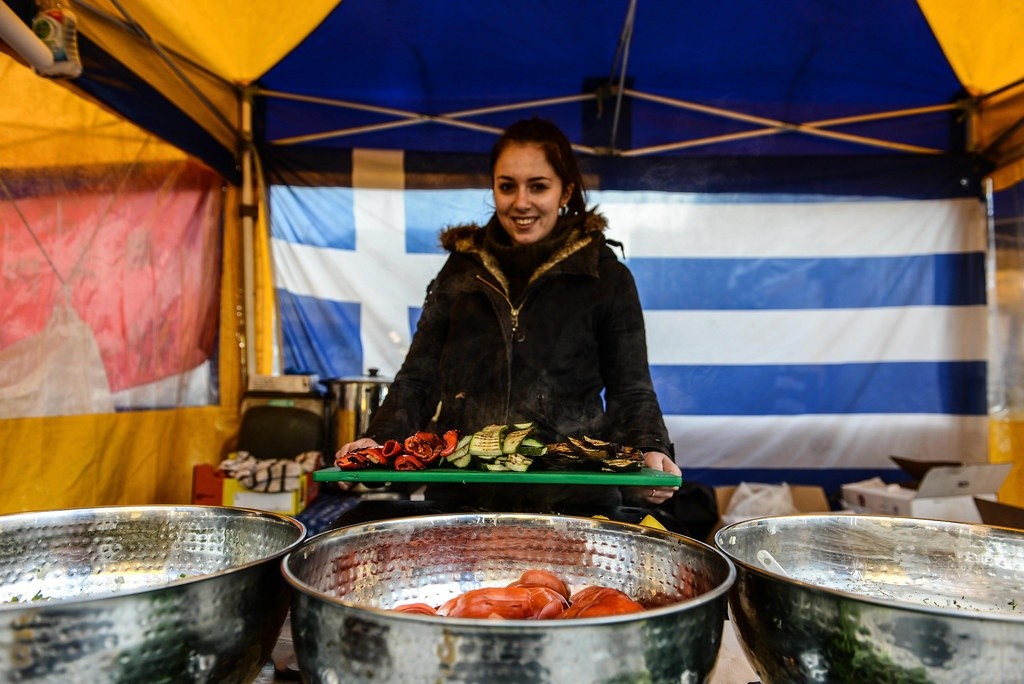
[332,115,683,521]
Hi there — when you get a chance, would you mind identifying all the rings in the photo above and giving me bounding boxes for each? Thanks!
[650,490,655,498]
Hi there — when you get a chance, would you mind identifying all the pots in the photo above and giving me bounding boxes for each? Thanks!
[317,368,394,464]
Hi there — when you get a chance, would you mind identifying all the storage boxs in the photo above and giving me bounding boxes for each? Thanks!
[713,483,831,528]
[193,372,340,515]
[837,455,1013,524]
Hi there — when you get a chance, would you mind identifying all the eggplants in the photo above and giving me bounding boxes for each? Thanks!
[541,436,644,474]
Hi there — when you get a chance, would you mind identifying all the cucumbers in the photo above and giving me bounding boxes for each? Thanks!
[439,422,549,472]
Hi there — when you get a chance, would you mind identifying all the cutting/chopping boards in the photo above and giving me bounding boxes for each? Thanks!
[313,463,683,487]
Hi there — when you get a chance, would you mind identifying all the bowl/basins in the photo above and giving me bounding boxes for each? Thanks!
[281,512,736,684]
[713,512,1024,684]
[0,502,307,683]
